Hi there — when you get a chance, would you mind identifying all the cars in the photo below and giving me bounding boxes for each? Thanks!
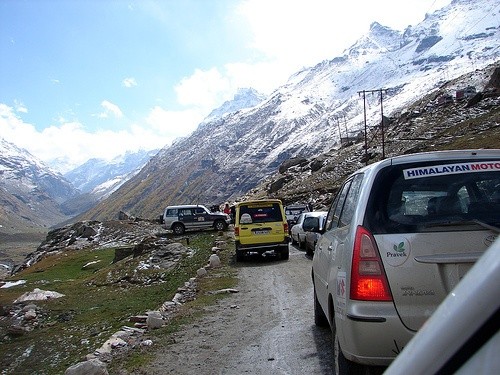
[306,214,326,255]
[310,144,500,375]
[288,212,318,247]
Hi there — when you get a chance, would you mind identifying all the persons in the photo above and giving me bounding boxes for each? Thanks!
[224,203,230,214]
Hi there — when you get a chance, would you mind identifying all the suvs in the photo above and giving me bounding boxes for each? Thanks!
[159,204,232,234]
[234,198,287,258]
[284,205,308,234]
